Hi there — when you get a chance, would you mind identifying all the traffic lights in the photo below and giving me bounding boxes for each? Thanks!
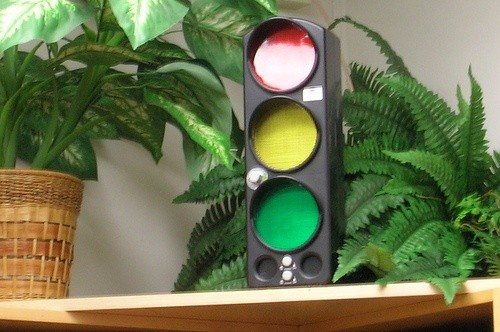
[243,17,346,287]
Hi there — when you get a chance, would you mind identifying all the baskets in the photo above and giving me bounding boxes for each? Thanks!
[0,169,85,298]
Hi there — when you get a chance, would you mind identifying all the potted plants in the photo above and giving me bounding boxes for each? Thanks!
[0,0,278,299]
